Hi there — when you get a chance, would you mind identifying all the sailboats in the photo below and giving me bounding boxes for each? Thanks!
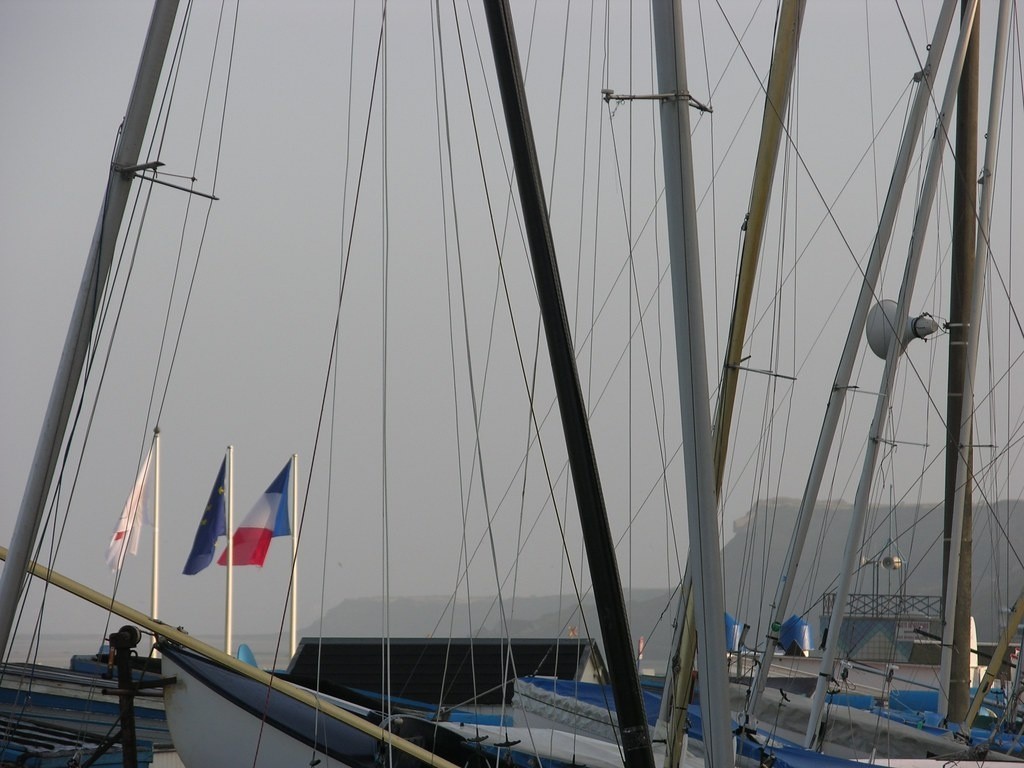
[0,0,1024,767]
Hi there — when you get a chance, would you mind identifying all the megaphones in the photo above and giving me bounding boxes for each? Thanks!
[866,300,938,360]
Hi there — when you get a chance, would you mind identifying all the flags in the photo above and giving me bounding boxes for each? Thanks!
[217,457,291,567]
[105,447,153,573]
[182,454,226,575]
